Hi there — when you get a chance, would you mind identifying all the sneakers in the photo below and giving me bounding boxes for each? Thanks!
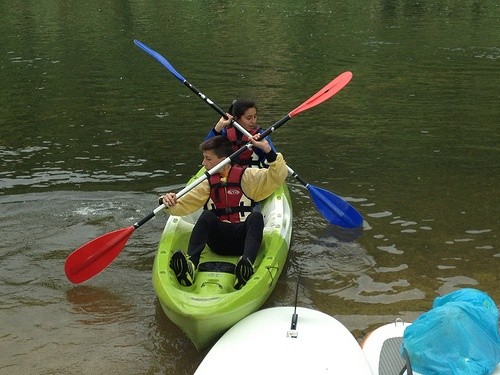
[169,250,196,287]
[233,255,254,290]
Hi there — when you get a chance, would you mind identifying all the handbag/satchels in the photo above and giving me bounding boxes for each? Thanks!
[400,289,500,375]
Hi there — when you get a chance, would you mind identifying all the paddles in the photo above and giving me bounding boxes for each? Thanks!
[64,72,354,284]
[132,39,364,228]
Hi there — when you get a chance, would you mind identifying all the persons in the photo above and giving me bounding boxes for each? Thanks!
[203,100,279,166]
[158,133,288,290]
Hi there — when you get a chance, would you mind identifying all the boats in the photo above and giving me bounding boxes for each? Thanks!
[194,305,372,375]
[150,166,294,350]
[360,322,422,375]
